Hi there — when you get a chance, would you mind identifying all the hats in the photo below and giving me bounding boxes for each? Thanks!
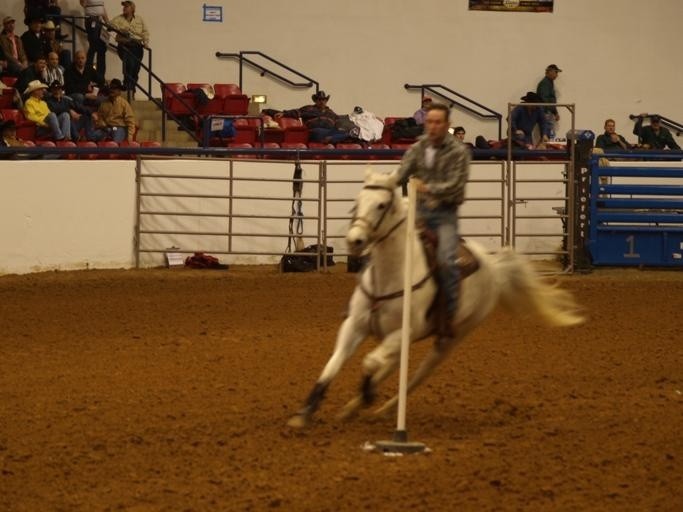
[2,16,15,21]
[547,64,561,73]
[42,21,59,29]
[311,92,330,102]
[105,79,126,91]
[23,80,48,95]
[49,79,64,91]
[520,91,542,103]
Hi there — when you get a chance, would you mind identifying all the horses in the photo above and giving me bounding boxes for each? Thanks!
[284,164,590,430]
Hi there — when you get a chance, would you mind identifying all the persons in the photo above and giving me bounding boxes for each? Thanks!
[537,64,563,141]
[414,98,433,127]
[453,127,474,149]
[506,92,549,151]
[275,91,338,141]
[0,0,148,160]
[595,119,632,150]
[632,114,681,151]
[390,103,468,353]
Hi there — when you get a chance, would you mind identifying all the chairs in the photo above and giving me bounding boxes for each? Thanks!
[2,67,166,157]
[153,69,572,163]
